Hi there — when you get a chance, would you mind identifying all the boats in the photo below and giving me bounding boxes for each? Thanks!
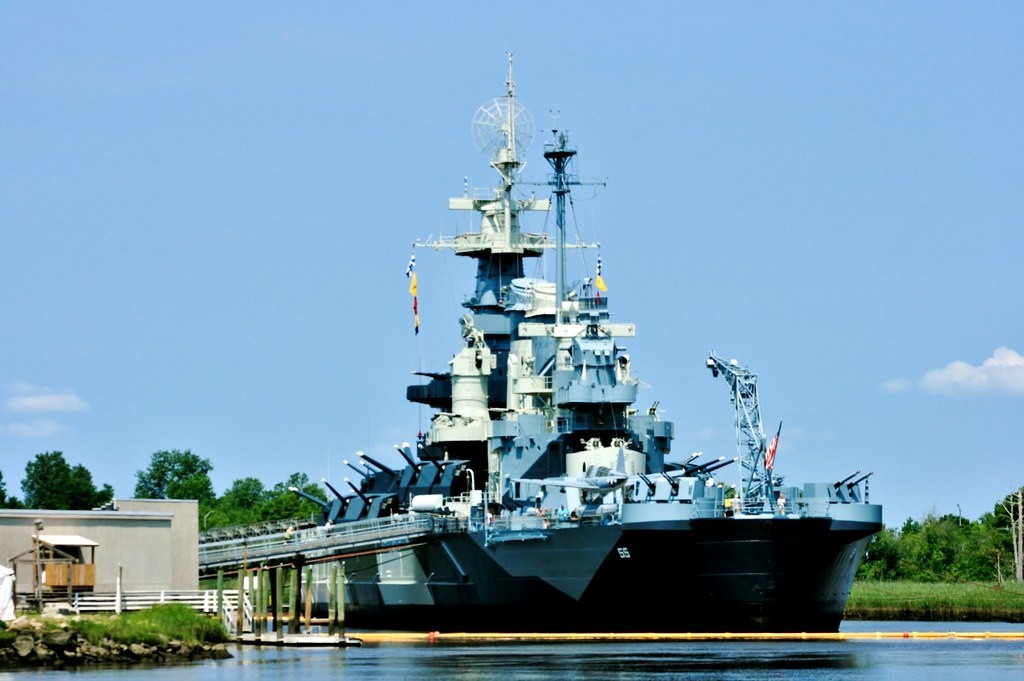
[286,50,885,642]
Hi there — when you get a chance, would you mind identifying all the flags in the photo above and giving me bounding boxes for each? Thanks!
[595,275,607,291]
[763,434,779,470]
[406,251,416,276]
[411,275,419,335]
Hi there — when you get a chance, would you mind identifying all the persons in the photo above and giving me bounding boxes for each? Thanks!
[283,525,294,544]
[486,504,581,529]
[325,518,333,537]
[777,492,786,516]
